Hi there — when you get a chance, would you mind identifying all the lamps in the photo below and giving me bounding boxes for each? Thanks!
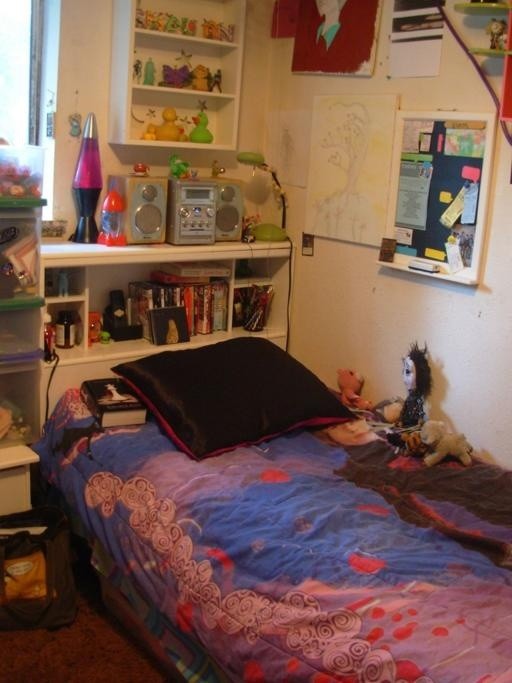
[237,151,291,242]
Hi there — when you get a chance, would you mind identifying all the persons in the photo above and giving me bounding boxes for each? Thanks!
[245,282,275,330]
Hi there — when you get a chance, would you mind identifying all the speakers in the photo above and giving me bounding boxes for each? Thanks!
[108,174,168,244]
[202,177,243,242]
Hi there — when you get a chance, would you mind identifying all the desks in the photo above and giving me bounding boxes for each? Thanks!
[0,445,41,516]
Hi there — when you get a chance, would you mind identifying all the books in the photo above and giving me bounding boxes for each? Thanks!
[79,379,148,429]
[128,264,232,345]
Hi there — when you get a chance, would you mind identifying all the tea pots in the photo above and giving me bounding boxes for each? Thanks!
[133,160,150,174]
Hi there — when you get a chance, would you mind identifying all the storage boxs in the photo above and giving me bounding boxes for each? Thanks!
[0,146,47,449]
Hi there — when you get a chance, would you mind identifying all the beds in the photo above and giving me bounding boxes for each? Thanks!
[30,386,512,683]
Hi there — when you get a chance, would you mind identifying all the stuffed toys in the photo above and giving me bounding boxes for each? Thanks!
[167,152,199,178]
[336,347,473,467]
[483,18,507,49]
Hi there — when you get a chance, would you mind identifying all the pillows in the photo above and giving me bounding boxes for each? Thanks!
[111,336,362,462]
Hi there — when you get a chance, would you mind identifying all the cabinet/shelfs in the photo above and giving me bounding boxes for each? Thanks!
[40,235,296,433]
[107,0,247,151]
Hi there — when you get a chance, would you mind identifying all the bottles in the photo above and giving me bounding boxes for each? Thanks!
[144,56,155,85]
[55,311,76,347]
[45,325,55,357]
[214,304,224,329]
[55,271,68,297]
[111,289,127,326]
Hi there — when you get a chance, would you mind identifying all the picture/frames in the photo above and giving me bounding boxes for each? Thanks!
[374,109,498,288]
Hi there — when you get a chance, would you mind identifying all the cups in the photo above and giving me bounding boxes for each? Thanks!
[244,302,265,332]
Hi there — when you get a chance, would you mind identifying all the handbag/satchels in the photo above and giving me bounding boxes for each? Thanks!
[0,507,79,630]
